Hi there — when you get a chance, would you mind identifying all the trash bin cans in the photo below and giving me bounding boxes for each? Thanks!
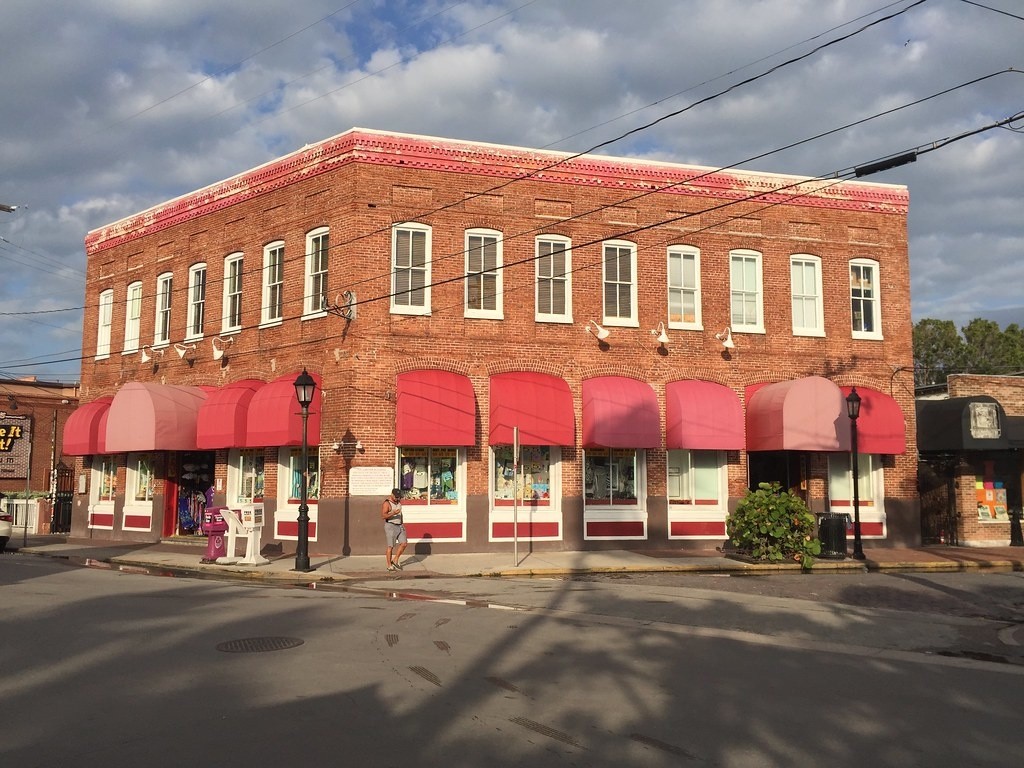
[814,512,850,560]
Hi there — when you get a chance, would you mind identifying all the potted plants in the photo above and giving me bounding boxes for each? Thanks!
[725,482,821,569]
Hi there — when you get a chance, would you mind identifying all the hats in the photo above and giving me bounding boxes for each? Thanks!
[391,488,401,498]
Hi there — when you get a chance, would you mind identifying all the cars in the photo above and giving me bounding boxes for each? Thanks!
[0,508,13,550]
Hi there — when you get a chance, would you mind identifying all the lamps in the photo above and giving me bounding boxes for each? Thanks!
[174,343,196,359]
[585,320,610,339]
[716,327,735,349]
[651,321,670,343]
[141,344,164,364]
[211,337,233,360]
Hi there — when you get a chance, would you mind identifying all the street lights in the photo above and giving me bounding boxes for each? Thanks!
[846,386,866,560]
[289,367,316,573]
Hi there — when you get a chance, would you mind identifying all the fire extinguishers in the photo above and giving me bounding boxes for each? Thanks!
[939,527,945,545]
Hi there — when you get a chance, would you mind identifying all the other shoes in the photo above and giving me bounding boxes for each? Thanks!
[386,566,398,572]
[390,560,403,571]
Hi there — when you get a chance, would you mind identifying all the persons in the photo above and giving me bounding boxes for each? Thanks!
[382,488,408,572]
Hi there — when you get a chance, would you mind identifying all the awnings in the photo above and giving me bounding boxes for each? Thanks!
[395,370,476,447]
[489,372,575,446]
[748,376,851,451]
[917,395,1024,451]
[196,379,267,449]
[98,406,122,455]
[665,381,745,451]
[844,387,906,454]
[63,401,111,456]
[244,372,321,447]
[104,381,218,453]
[582,377,660,448]
